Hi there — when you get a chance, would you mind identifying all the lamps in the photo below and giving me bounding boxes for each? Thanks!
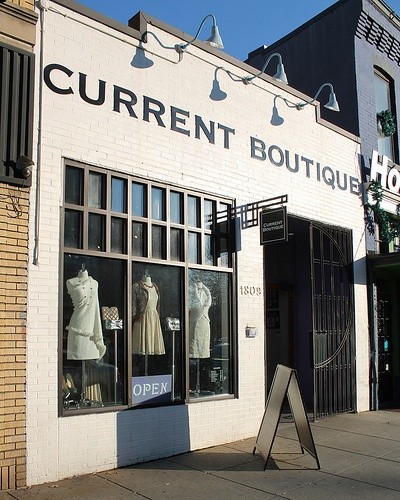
[244,53,288,84]
[176,14,225,52]
[296,83,341,112]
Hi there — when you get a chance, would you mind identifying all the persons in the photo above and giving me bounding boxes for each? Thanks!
[132,275,166,356]
[187,280,213,358]
[65,269,107,361]
